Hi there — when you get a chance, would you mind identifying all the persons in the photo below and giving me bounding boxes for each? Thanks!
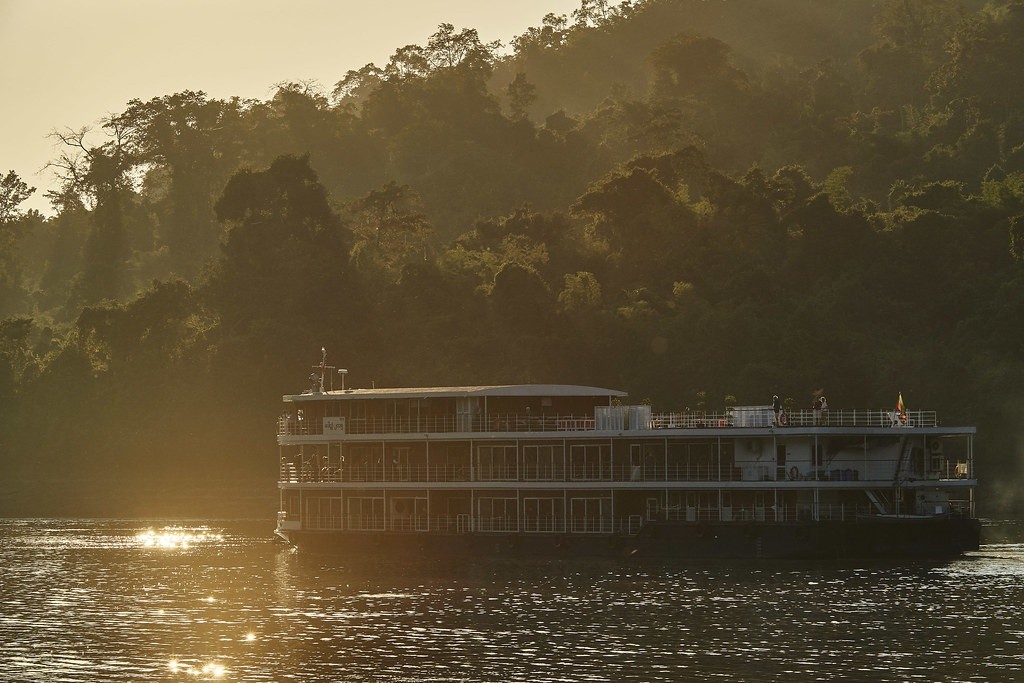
[772,396,782,426]
[813,396,828,426]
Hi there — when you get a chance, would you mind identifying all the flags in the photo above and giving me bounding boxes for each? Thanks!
[898,393,908,419]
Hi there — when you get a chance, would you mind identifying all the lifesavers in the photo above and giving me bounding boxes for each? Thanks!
[778,412,790,428]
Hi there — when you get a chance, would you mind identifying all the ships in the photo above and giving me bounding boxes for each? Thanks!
[273,377,983,557]
